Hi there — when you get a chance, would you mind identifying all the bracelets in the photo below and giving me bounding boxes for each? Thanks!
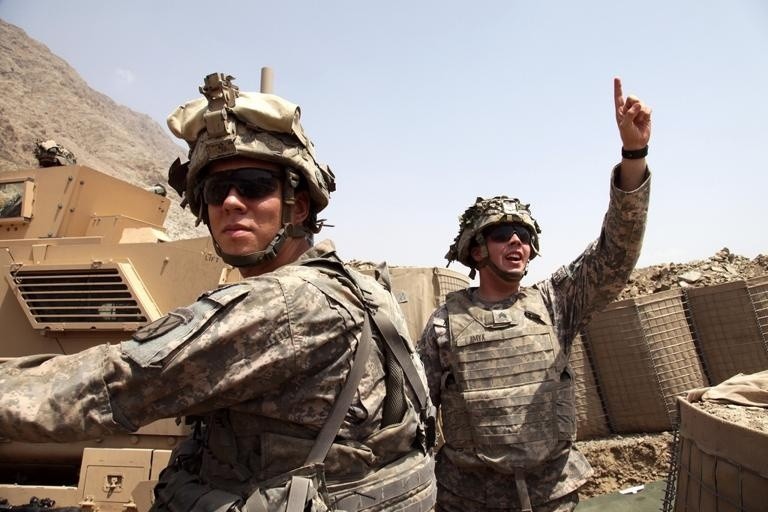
[621,145,649,159]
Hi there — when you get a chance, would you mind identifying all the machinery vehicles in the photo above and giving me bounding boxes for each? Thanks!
[0,139,245,512]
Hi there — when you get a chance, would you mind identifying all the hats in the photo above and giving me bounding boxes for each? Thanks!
[455,195,540,267]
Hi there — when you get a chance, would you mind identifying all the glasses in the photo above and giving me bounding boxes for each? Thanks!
[198,164,282,204]
[481,223,533,244]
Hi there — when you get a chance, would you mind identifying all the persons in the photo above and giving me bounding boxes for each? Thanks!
[413,77,653,511]
[0,70,440,511]
[1,140,77,218]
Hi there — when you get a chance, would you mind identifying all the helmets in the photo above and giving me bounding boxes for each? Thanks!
[187,121,331,212]
